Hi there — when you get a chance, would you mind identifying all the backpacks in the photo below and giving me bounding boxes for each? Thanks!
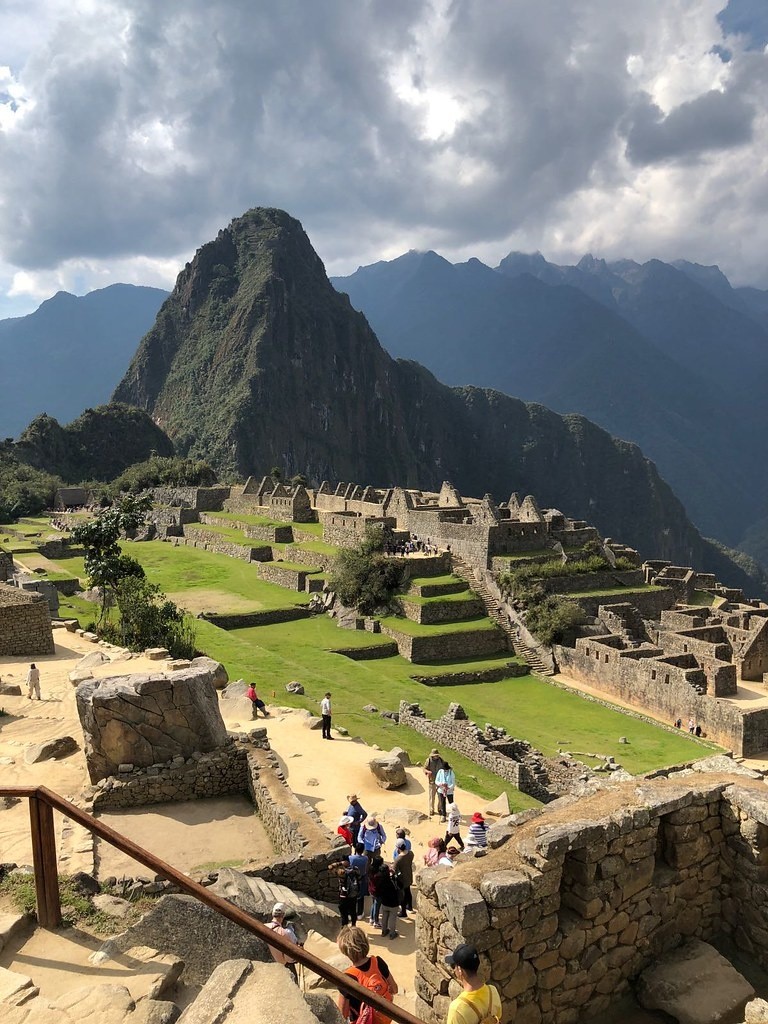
[457,985,503,1024]
[338,866,360,899]
[341,956,393,1024]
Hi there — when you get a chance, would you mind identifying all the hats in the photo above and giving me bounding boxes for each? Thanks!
[272,902,286,916]
[446,803,460,814]
[439,762,451,769]
[347,794,360,802]
[429,749,440,756]
[471,812,485,822]
[365,817,377,830]
[445,944,478,968]
[428,837,446,849]
[447,846,460,855]
[340,816,354,826]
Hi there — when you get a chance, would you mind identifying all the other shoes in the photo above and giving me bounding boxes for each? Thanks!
[382,929,390,936]
[327,737,334,740]
[370,919,376,925]
[374,922,382,928]
[390,932,400,939]
[441,816,446,822]
[358,912,365,919]
[323,736,327,738]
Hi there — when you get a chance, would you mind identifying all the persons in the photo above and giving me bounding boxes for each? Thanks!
[421,748,446,816]
[247,682,270,718]
[366,855,395,929]
[328,854,363,929]
[445,943,503,1024]
[25,663,42,700]
[391,838,415,917]
[347,842,370,920]
[337,792,490,867]
[434,761,455,824]
[385,529,452,558]
[374,864,404,940]
[336,926,398,1024]
[320,691,334,740]
[261,902,299,989]
